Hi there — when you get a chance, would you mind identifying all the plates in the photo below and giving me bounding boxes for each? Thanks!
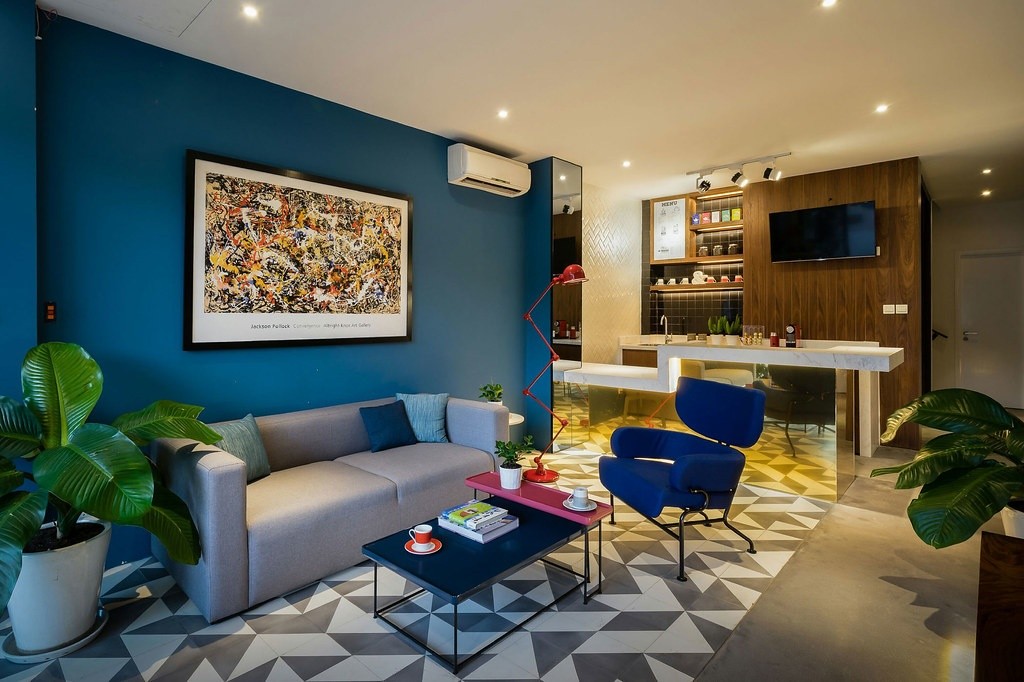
[563,499,597,512]
[404,538,442,555]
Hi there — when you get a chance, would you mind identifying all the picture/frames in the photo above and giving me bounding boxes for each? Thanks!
[182,148,414,351]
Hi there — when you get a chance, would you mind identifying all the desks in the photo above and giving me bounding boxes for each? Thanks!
[508,409,524,426]
[360,495,590,675]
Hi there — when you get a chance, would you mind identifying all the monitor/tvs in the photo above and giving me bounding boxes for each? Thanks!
[769,200,877,264]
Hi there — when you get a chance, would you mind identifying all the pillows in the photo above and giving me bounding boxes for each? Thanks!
[396,392,450,445]
[206,412,272,481]
[359,400,420,454]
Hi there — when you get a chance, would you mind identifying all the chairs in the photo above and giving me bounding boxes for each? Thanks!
[599,376,766,583]
[753,364,836,459]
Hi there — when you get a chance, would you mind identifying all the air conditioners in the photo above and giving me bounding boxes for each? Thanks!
[447,143,532,199]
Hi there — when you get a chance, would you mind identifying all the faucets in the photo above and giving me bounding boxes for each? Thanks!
[660,315,672,344]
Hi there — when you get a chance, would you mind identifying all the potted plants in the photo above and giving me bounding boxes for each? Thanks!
[477,381,504,406]
[723,314,741,346]
[707,315,727,346]
[0,341,224,665]
[870,386,1024,549]
[493,434,535,490]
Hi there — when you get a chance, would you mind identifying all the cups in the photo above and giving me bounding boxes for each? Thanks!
[721,276,730,282]
[409,525,433,549]
[707,277,716,283]
[680,278,688,284]
[699,246,709,256]
[567,488,589,509]
[687,332,696,343]
[656,278,664,285]
[697,333,707,342]
[713,245,723,256]
[667,278,675,285]
[728,243,739,254]
[735,274,744,283]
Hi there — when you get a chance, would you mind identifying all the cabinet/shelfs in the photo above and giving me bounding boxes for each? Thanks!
[650,190,744,291]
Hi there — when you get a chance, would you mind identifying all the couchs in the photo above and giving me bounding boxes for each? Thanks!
[150,392,510,625]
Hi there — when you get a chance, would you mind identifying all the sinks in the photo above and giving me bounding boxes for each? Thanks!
[637,343,665,347]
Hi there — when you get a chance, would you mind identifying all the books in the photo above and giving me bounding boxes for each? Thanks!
[436,514,519,544]
[440,499,508,531]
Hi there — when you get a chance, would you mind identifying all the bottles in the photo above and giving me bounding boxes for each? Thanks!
[770,332,779,347]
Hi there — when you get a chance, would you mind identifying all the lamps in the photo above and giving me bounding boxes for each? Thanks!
[730,164,748,187]
[563,203,574,215]
[695,170,711,195]
[524,265,589,482]
[762,159,781,182]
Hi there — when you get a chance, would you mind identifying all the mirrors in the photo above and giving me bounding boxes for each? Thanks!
[550,158,582,344]
